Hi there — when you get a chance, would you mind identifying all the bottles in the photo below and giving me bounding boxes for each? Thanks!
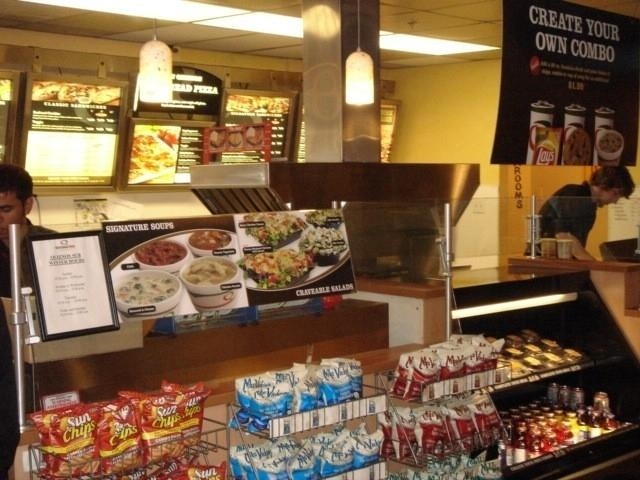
[540,238,573,259]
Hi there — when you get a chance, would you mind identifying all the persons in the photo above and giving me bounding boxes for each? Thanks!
[522,164,636,256]
[0,298,22,480]
[0,164,62,297]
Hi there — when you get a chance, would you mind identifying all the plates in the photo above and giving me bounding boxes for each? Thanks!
[235,212,352,296]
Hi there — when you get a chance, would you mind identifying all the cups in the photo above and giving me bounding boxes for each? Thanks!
[526,101,555,166]
[595,107,615,129]
[595,129,624,166]
[565,103,586,131]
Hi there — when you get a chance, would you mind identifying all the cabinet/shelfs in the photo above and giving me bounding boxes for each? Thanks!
[226,384,409,480]
[28,416,229,479]
[450,260,640,479]
[374,359,513,479]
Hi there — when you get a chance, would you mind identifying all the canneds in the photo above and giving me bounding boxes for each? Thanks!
[547,381,610,410]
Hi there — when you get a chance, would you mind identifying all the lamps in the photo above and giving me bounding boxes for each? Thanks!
[340,0,378,109]
[134,16,175,104]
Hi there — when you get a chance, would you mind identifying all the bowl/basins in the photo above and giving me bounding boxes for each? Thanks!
[111,229,241,324]
